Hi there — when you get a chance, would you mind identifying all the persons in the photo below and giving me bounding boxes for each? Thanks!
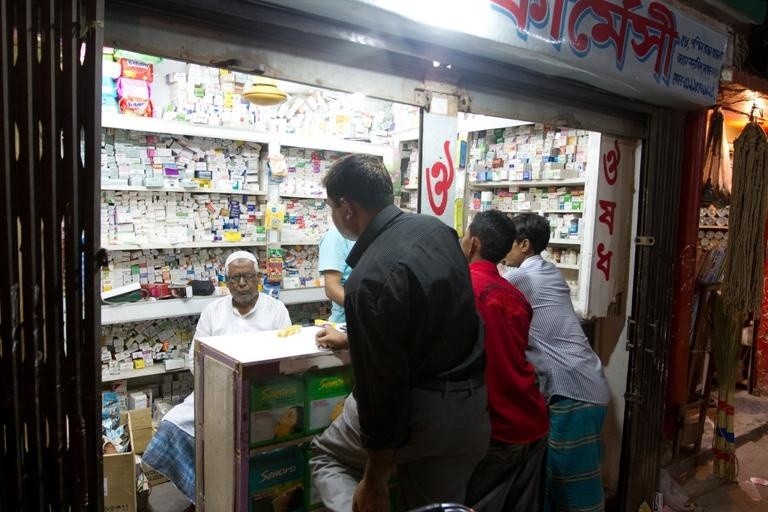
[495,211,614,511]
[452,206,555,512]
[312,229,360,325]
[304,151,493,511]
[140,248,295,511]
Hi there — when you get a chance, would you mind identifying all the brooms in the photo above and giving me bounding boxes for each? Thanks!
[703,291,741,479]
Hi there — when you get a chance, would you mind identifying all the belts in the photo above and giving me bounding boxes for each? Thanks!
[421,379,485,393]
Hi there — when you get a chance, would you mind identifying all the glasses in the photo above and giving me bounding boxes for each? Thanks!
[227,273,258,281]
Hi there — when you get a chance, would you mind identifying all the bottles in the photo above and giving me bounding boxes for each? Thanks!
[472,192,493,210]
[546,246,580,265]
[570,281,577,295]
